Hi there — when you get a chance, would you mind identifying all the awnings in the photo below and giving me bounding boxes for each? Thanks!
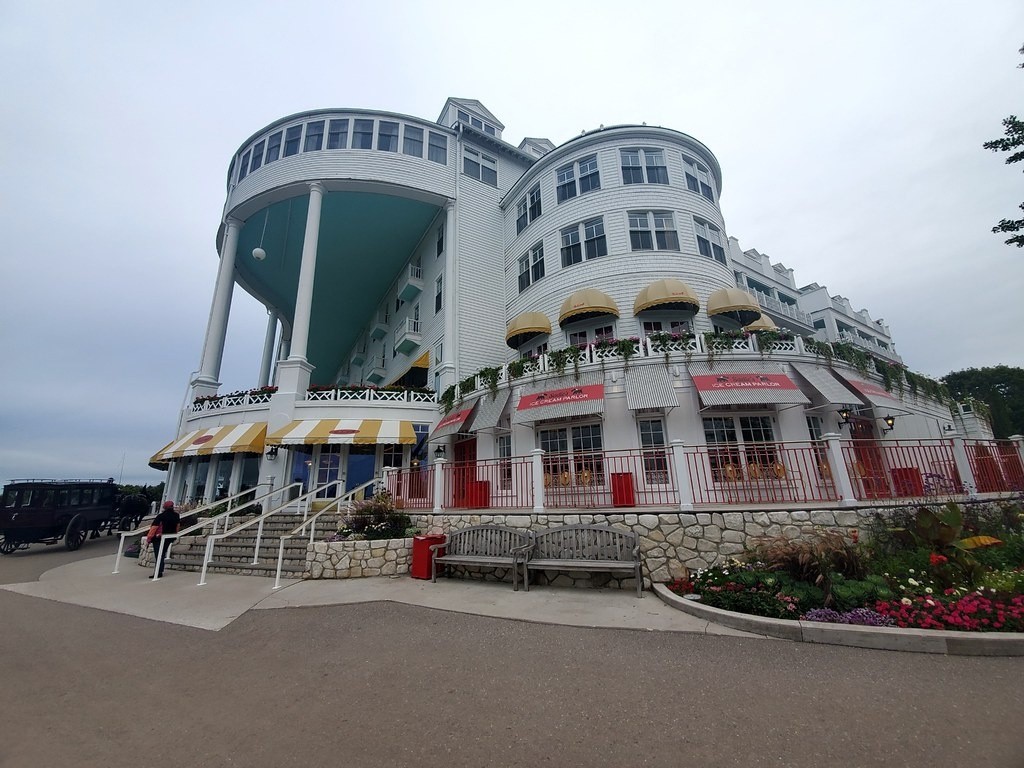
[264,420,418,445]
[624,363,680,410]
[147,423,266,471]
[512,371,605,425]
[790,363,866,406]
[468,387,512,433]
[426,396,478,443]
[830,366,915,415]
[685,361,812,404]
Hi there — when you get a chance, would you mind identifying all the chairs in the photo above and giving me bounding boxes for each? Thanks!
[544,468,596,508]
[723,459,889,504]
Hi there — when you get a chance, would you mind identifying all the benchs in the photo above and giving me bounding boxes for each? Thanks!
[517,525,644,598]
[429,525,530,592]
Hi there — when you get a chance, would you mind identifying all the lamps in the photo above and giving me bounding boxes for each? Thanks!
[411,457,421,467]
[266,447,278,460]
[943,421,955,434]
[434,444,445,457]
[882,413,895,436]
[252,207,270,260]
[837,406,852,430]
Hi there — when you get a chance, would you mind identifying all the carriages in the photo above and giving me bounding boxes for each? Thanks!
[0,478,155,555]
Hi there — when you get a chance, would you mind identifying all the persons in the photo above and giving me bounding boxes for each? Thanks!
[145,500,181,579]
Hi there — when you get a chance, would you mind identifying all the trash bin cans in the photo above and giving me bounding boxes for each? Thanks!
[467,481,489,509]
[412,535,446,580]
[610,472,635,507]
[891,467,925,497]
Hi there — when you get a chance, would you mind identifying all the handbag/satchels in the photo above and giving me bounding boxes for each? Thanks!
[147,525,159,538]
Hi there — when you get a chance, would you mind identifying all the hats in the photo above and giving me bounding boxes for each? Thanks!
[163,501,175,508]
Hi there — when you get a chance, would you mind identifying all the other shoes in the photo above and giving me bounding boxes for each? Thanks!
[149,570,162,578]
